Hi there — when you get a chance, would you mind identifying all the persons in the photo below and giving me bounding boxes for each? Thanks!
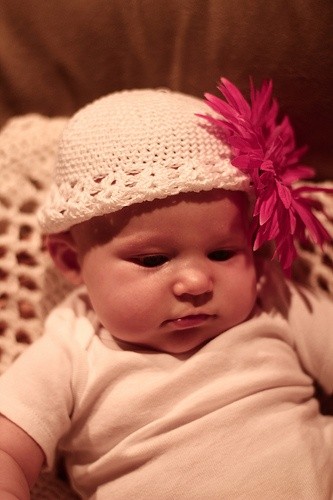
[0,77,333,500]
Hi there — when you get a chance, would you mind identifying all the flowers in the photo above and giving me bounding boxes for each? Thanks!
[193,77,333,270]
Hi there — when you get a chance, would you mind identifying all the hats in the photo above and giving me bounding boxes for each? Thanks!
[36,79,332,278]
